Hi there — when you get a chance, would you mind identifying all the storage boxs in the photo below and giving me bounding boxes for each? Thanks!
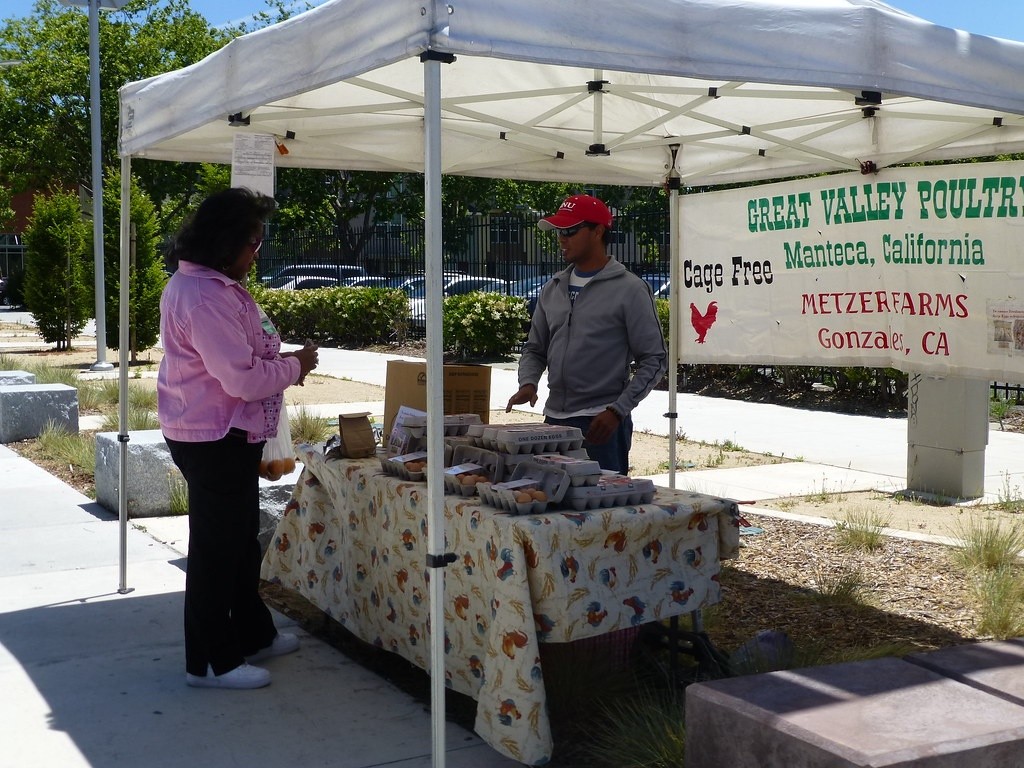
[382,360,491,449]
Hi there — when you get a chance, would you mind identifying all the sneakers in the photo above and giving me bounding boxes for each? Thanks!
[186,659,270,690]
[243,633,298,660]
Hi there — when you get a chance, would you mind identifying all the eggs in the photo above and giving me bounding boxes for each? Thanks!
[260,458,294,481]
[403,459,546,503]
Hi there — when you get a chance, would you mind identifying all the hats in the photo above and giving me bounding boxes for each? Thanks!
[538,195,614,231]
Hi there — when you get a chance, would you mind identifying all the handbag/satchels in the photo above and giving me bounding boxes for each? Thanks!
[261,395,296,481]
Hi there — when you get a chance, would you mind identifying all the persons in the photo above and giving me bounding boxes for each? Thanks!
[505,195,669,477]
[158,187,320,689]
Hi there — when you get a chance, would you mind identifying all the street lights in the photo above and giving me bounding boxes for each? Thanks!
[55,0,134,369]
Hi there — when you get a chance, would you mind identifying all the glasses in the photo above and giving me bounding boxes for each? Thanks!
[248,239,262,254]
[556,224,590,238]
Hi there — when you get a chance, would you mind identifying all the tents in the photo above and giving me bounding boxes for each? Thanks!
[116,0,1024,767]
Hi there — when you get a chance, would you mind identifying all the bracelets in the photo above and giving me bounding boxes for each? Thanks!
[605,406,623,421]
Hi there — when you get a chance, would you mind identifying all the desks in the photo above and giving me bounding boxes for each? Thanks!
[259,445,741,765]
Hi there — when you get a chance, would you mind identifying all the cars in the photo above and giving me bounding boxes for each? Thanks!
[639,275,671,300]
[246,262,553,333]
